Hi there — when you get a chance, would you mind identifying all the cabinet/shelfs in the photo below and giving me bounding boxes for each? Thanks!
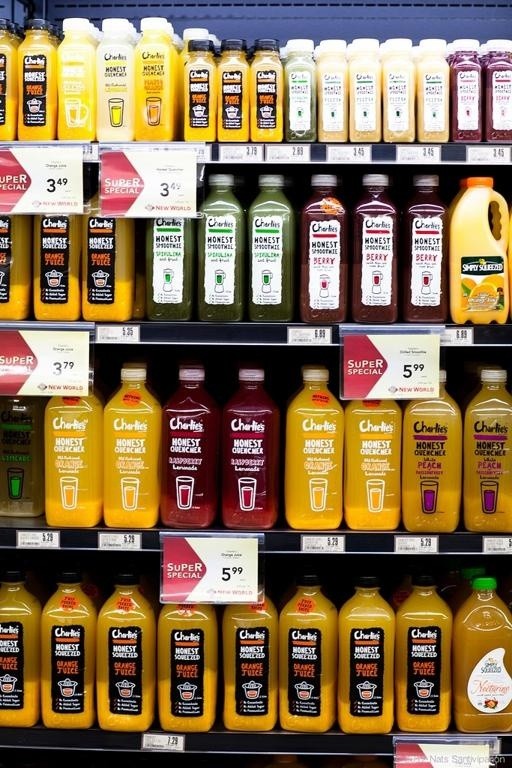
[0,1,512,767]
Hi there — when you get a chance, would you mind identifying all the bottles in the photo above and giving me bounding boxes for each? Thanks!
[222,368,281,530]
[453,574,512,734]
[143,216,194,322]
[32,214,83,322]
[285,366,343,532]
[485,42,512,146]
[44,398,102,528]
[1,216,32,321]
[221,591,280,730]
[350,173,400,324]
[158,604,219,731]
[450,175,510,326]
[195,173,244,323]
[248,39,284,145]
[320,39,352,145]
[82,182,137,321]
[133,17,179,139]
[245,174,295,324]
[1,396,43,519]
[1,569,41,730]
[298,172,350,322]
[462,371,510,534]
[450,38,482,145]
[281,37,316,142]
[219,37,250,143]
[382,38,418,144]
[16,17,58,143]
[278,571,339,729]
[56,17,100,143]
[394,575,452,734]
[102,366,159,530]
[401,370,463,535]
[339,571,395,729]
[97,566,156,733]
[97,17,134,145]
[183,39,219,142]
[159,363,222,531]
[42,571,97,731]
[0,18,19,140]
[402,174,450,322]
[343,399,402,530]
[417,39,451,146]
[349,37,381,143]
